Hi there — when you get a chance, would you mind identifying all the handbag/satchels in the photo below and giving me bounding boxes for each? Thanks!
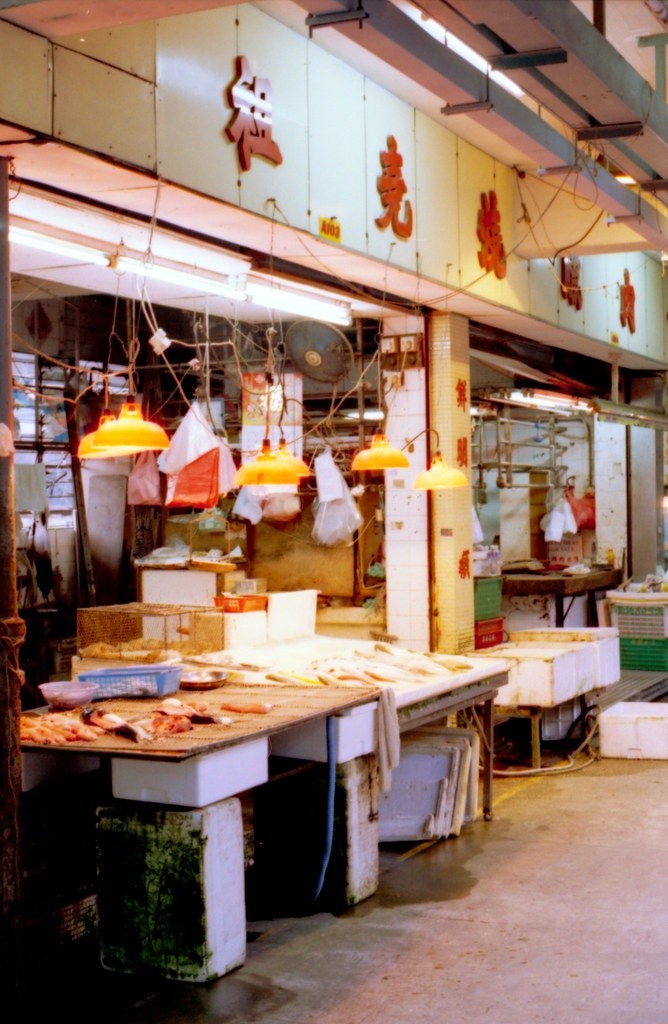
[564,485,595,530]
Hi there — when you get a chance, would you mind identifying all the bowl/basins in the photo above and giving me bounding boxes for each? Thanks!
[38,681,100,709]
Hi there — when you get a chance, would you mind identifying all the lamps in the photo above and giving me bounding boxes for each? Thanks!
[351,350,410,470]
[233,384,310,486]
[400,428,470,489]
[77,371,170,459]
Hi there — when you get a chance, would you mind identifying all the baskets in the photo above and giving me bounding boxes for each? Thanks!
[214,594,268,613]
[236,578,268,594]
[545,533,583,569]
[77,666,183,700]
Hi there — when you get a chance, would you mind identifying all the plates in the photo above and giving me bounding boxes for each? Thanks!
[180,671,231,687]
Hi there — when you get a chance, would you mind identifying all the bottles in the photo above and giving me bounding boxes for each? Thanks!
[487,545,501,576]
[607,550,614,565]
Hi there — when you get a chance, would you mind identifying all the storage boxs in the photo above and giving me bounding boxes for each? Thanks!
[509,627,621,688]
[474,577,504,649]
[467,642,594,708]
[598,701,668,759]
[268,701,379,765]
[111,736,269,808]
[606,590,668,672]
